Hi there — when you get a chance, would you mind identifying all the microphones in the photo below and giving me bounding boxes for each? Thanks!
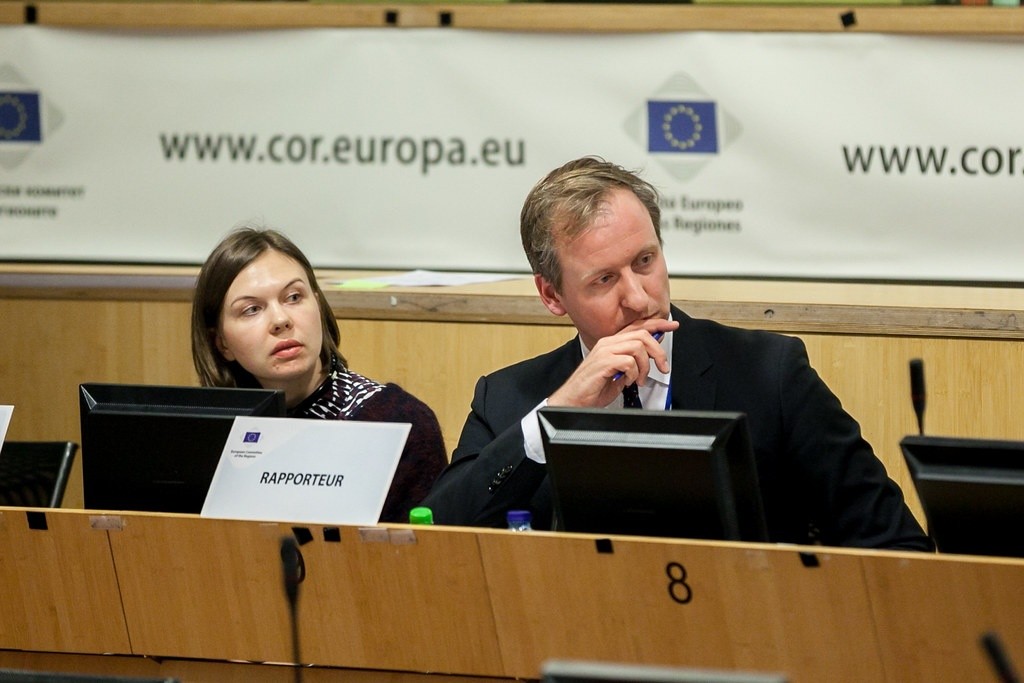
[910,359,926,435]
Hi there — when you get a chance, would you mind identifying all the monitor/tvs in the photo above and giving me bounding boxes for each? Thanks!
[78,383,289,515]
[900,435,1024,558]
[537,406,767,544]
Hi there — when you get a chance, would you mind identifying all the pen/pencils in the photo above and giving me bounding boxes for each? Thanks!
[612,332,663,381]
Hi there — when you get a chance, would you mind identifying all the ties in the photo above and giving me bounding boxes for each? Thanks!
[622,382,643,411]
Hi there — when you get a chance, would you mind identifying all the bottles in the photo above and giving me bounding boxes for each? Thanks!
[410,507,434,526]
[508,510,532,531]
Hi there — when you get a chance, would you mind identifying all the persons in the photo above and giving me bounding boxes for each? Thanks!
[189,226,450,523]
[418,158,938,557]
